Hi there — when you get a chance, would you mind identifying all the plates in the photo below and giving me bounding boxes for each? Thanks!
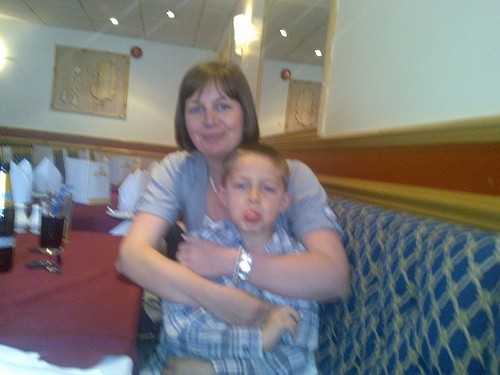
[106,209,134,219]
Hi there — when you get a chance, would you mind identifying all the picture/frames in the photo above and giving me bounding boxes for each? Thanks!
[51,44,131,123]
[282,76,322,132]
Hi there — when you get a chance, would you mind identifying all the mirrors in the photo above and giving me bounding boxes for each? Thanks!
[255,0,337,145]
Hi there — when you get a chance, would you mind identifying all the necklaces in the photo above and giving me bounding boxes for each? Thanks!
[209,176,219,194]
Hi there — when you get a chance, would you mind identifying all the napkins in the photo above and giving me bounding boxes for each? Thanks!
[32,157,65,196]
[115,167,146,213]
[10,156,32,204]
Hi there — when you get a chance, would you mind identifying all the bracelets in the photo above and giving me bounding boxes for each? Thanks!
[233,245,253,284]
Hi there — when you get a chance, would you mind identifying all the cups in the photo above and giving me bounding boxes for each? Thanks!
[37,195,66,253]
[48,191,73,243]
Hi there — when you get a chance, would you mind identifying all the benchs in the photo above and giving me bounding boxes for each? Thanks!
[1,136,500,375]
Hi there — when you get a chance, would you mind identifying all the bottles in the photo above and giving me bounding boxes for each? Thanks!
[13,203,29,233]
[29,205,41,235]
[0,161,14,273]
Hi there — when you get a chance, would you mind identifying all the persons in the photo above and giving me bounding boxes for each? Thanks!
[115,61,351,325]
[137,143,319,375]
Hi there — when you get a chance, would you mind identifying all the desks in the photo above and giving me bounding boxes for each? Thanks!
[0,232,144,374]
